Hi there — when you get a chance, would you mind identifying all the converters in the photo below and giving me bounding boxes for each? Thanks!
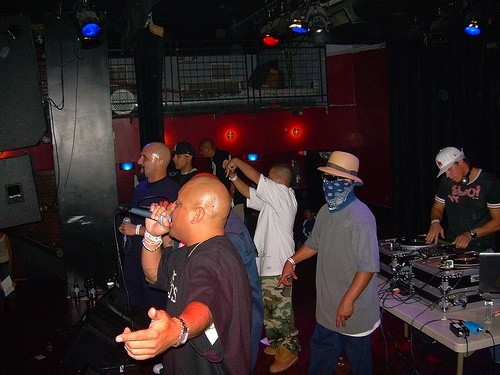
[451,322,470,337]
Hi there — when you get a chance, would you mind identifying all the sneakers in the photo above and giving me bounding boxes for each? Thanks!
[264,347,298,371]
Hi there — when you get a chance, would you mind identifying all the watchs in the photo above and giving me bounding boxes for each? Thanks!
[172,316,189,347]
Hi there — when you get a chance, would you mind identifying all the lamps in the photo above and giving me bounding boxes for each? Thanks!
[259,0,329,48]
[74,0,102,43]
[407,0,485,43]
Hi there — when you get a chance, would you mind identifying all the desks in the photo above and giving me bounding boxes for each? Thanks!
[376,276,500,375]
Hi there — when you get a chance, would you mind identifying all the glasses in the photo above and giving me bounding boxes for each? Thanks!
[321,172,348,181]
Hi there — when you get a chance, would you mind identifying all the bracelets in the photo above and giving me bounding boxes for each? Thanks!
[144,230,162,242]
[288,258,295,265]
[9,261,13,263]
[144,237,161,245]
[431,219,440,224]
[142,239,161,252]
[228,174,237,181]
[469,229,477,239]
[135,225,141,235]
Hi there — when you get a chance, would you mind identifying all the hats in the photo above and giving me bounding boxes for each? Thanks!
[435,147,465,180]
[191,173,235,201]
[317,151,363,186]
[172,142,196,159]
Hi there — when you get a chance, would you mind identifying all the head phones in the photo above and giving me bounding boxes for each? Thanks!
[460,165,473,185]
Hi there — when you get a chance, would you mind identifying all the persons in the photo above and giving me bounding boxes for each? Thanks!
[116,140,270,375]
[302,206,317,238]
[222,158,300,375]
[0,232,17,313]
[278,151,381,375]
[425,147,500,250]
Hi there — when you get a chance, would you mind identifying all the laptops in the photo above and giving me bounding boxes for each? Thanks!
[477,253,500,299]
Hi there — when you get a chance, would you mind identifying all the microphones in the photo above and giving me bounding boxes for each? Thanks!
[119,206,172,225]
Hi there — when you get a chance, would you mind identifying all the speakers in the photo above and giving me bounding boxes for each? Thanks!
[0,15,47,153]
[61,284,153,375]
[0,155,42,230]
